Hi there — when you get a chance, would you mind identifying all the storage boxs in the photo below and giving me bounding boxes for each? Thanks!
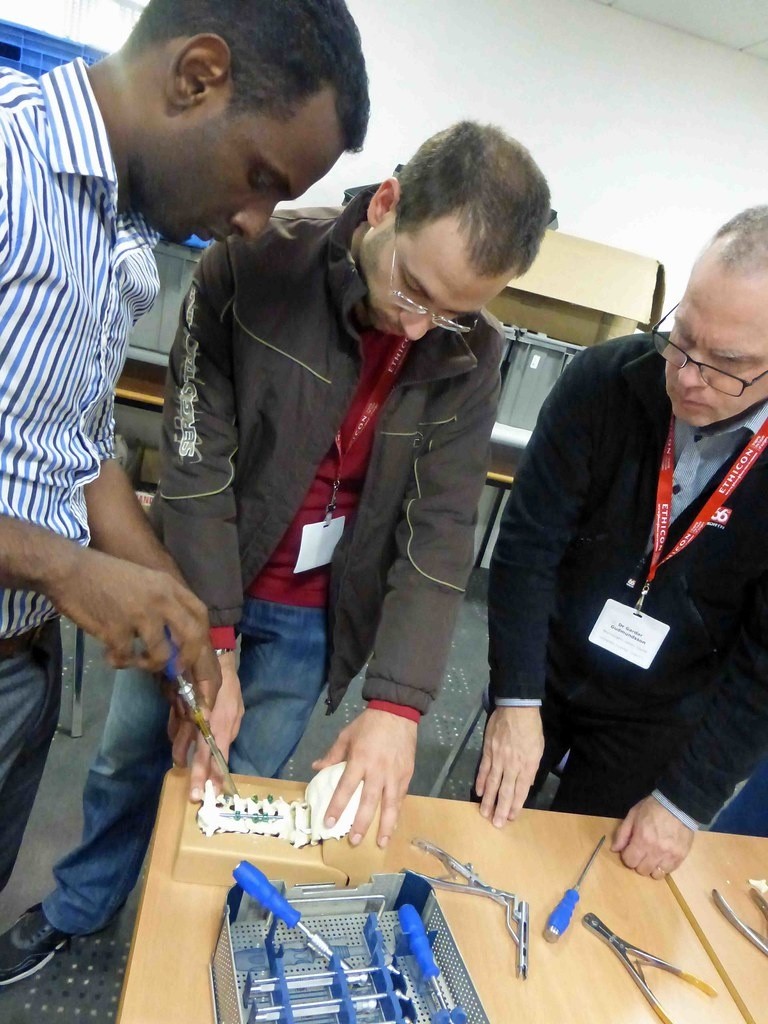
[486,227,666,348]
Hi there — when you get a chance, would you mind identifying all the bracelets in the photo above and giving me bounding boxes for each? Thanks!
[214,648,230,655]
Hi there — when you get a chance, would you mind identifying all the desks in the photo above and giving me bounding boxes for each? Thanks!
[111,768,768,1024]
[113,376,513,571]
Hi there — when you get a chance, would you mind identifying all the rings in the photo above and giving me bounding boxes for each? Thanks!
[657,866,665,873]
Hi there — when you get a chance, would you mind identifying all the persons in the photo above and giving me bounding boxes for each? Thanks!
[469,206,768,881]
[1,121,553,993]
[1,0,369,903]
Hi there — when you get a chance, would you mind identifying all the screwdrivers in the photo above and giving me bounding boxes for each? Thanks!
[543,835,605,943]
[164,625,241,800]
[232,860,469,1024]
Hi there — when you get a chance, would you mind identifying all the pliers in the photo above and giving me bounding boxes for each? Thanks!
[711,888,768,958]
[399,836,530,980]
[582,912,719,1024]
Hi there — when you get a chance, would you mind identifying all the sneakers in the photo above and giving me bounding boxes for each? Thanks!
[0,903,77,985]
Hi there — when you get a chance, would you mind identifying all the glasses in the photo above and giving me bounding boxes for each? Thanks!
[388,204,483,334]
[652,301,768,396]
